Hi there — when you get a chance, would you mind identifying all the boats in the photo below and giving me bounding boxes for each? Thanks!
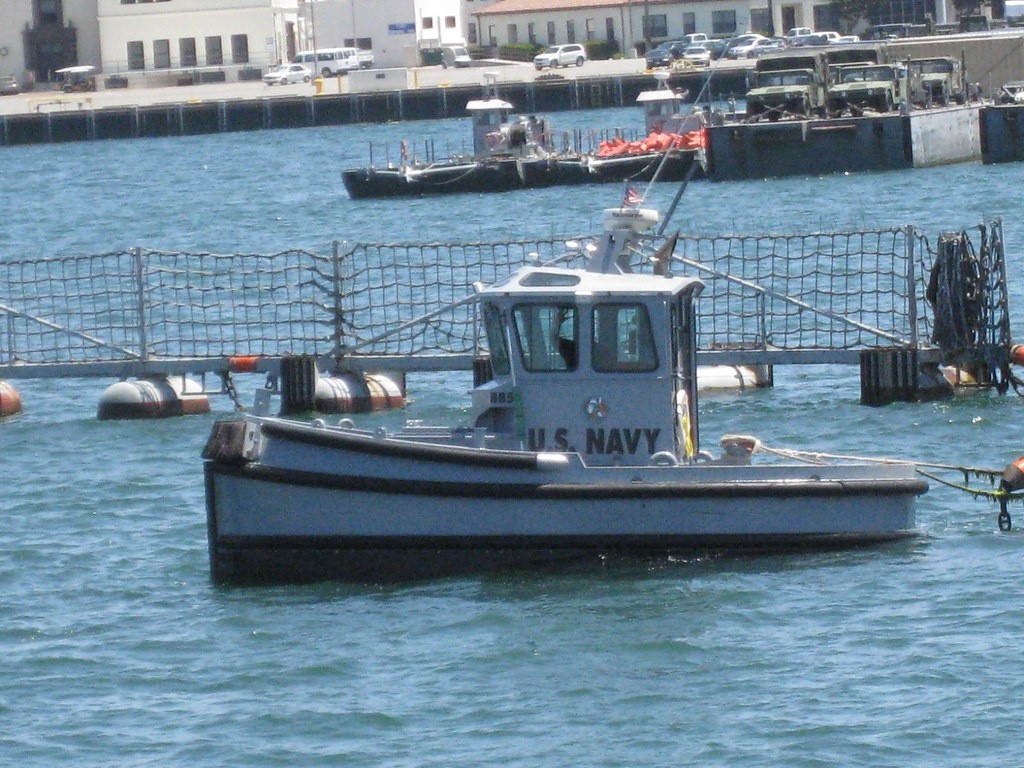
[199,175,931,571]
[339,112,709,200]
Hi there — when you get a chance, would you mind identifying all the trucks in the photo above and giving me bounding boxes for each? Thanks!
[900,54,969,107]
[827,42,904,114]
[866,14,1024,40]
[744,49,827,118]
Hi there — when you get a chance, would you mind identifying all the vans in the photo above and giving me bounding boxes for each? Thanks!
[291,45,377,79]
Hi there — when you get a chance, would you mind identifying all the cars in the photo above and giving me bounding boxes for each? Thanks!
[645,48,674,70]
[0,70,36,96]
[658,26,865,58]
[683,46,712,68]
[261,64,312,86]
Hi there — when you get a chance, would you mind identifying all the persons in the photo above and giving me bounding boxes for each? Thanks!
[674,99,729,151]
[924,14,932,35]
[550,303,617,372]
[509,106,548,158]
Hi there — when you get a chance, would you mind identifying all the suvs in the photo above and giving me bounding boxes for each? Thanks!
[533,43,588,71]
